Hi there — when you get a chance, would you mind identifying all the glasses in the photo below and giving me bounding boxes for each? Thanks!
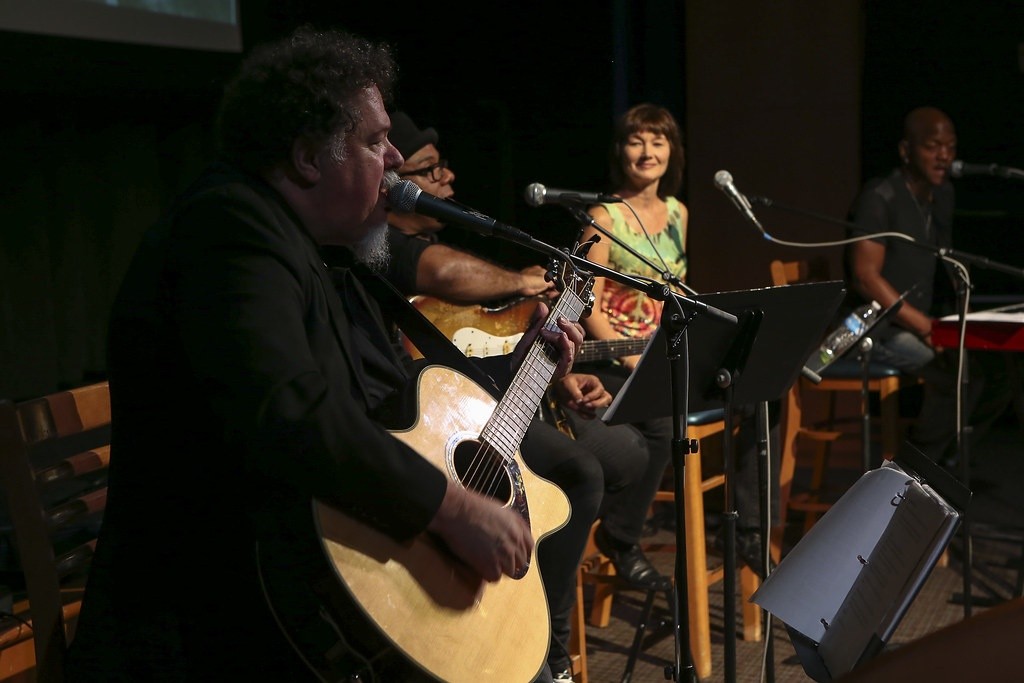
[397,158,450,181]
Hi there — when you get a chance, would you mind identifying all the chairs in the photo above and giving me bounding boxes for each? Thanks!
[1,383,111,683]
[548,258,925,683]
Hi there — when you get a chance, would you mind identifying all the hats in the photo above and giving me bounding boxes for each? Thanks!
[388,110,439,160]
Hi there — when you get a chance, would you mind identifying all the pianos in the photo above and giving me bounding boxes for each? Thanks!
[931,300,1023,352]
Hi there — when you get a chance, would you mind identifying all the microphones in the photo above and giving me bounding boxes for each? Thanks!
[388,179,532,243]
[714,170,765,236]
[949,161,1010,178]
[525,182,622,207]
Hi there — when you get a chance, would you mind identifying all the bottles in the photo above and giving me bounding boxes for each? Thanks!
[818,300,881,363]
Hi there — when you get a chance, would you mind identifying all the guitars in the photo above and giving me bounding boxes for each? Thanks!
[253,232,604,683]
[394,293,651,365]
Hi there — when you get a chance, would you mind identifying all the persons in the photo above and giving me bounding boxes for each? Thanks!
[67,31,585,683]
[578,104,777,586]
[824,106,1015,494]
[388,112,650,683]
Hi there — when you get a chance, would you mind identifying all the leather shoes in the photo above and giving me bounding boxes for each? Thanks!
[594,524,661,585]
[715,523,778,578]
